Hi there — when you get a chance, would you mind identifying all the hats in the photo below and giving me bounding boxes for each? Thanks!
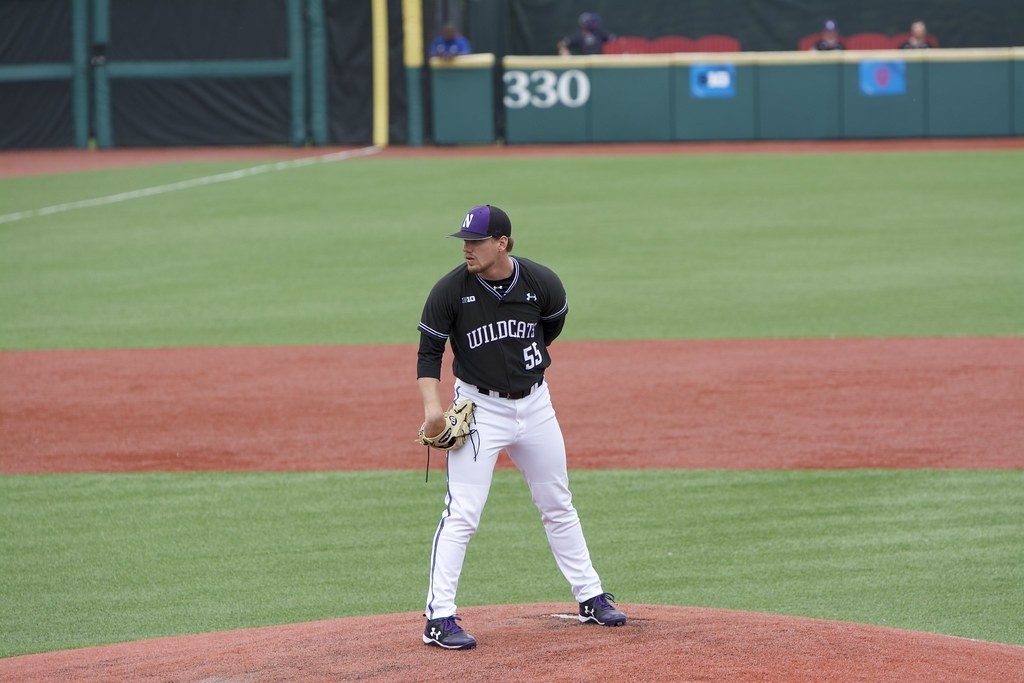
[444,205,511,241]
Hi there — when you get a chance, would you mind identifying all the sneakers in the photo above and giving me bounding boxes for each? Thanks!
[578,592,627,626]
[423,613,478,651]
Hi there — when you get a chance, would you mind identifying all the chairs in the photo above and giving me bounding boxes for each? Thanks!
[797,29,938,51]
[598,33,741,56]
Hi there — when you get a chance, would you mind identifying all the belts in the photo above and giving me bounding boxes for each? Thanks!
[462,378,545,401]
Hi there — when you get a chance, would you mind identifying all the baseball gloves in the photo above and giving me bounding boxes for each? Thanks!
[417,399,476,451]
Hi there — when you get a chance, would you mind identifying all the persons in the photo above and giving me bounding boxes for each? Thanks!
[416,205,628,649]
[558,13,613,55]
[808,20,847,50]
[429,22,471,61]
[899,21,934,49]
